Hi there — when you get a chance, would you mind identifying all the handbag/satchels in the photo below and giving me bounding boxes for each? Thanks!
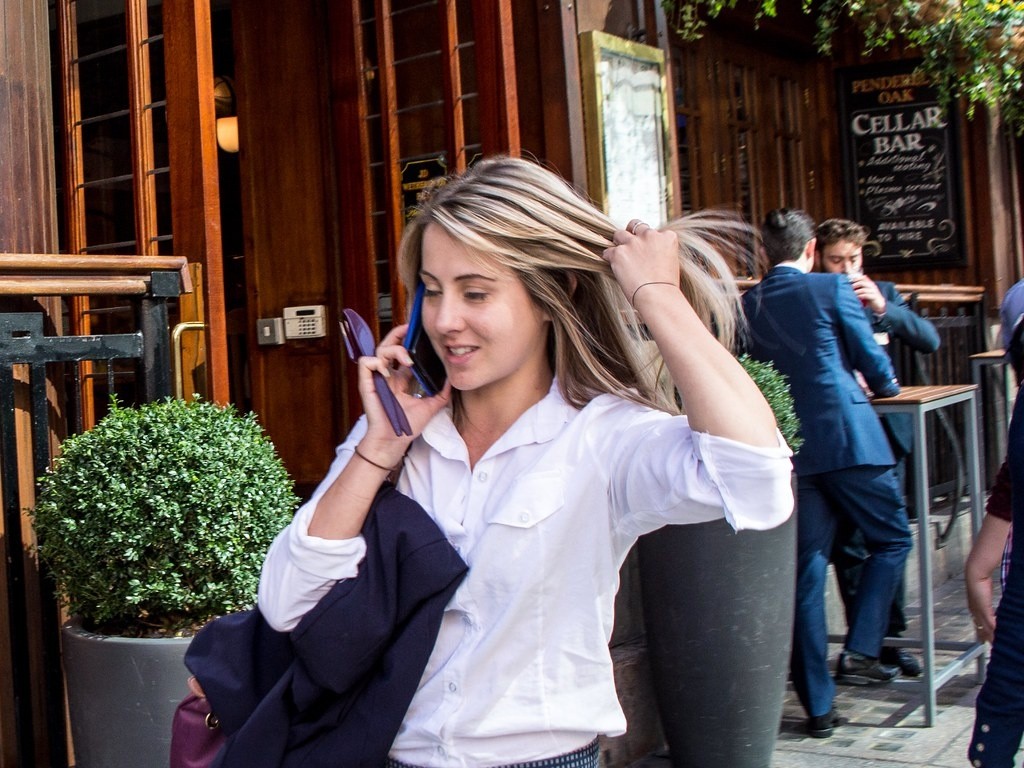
[168,676,227,768]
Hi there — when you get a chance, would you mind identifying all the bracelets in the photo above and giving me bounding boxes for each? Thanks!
[355,446,402,472]
[632,281,677,310]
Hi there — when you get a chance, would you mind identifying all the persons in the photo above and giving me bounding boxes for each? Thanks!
[818,218,940,676]
[735,207,913,737]
[258,156,795,768]
[964,278,1024,768]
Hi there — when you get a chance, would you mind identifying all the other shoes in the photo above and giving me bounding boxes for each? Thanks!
[883,643,922,674]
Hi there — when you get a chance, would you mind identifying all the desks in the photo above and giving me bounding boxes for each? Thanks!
[968,349,1015,514]
[827,384,984,725]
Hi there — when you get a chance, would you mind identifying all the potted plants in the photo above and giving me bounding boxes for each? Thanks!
[31,389,308,768]
[634,352,805,768]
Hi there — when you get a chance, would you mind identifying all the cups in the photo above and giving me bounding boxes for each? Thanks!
[845,268,870,307]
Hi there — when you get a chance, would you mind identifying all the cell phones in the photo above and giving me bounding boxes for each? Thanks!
[411,315,446,395]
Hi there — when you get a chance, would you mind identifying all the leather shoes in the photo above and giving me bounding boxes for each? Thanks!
[838,654,900,684]
[803,716,832,737]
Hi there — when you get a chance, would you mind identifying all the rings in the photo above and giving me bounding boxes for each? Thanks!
[977,625,983,630]
[632,221,650,235]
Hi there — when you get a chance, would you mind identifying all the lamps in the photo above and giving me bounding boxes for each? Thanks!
[217,116,241,153]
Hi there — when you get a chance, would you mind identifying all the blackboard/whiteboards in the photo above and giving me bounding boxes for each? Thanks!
[836,53,969,272]
